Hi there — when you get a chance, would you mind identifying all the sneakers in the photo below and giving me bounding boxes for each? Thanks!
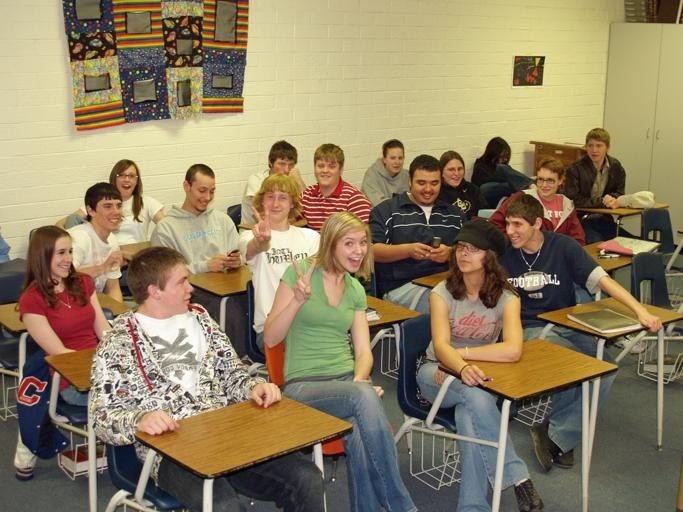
[553,448,575,469]
[530,416,553,471]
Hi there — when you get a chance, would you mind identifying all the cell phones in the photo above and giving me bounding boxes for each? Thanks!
[432,236,441,248]
[228,250,239,258]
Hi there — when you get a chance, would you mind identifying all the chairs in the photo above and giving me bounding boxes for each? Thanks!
[246,283,267,379]
[631,253,671,309]
[1,339,20,370]
[392,316,465,489]
[26,307,113,424]
[228,204,242,231]
[105,441,186,512]
[640,208,675,252]
[266,338,345,483]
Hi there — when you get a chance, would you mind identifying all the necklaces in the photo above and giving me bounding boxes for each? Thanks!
[52,287,75,309]
[519,241,544,272]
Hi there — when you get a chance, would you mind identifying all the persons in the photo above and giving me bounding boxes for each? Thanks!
[438,151,488,220]
[240,140,306,228]
[487,157,586,246]
[362,139,412,206]
[14,226,113,405]
[565,128,640,242]
[238,171,322,355]
[298,144,374,232]
[55,160,167,246]
[369,155,467,315]
[264,210,421,512]
[497,194,663,473]
[415,218,544,511]
[87,246,325,512]
[150,163,245,276]
[66,182,127,302]
[471,137,539,191]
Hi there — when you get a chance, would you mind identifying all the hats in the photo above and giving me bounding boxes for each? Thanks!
[453,219,506,256]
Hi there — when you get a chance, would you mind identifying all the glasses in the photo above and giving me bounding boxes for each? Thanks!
[115,172,138,179]
[532,177,556,185]
[454,243,480,252]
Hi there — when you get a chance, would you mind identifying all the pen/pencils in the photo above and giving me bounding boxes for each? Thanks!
[481,375,497,387]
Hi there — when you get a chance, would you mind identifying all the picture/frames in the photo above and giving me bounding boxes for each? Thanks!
[511,55,546,88]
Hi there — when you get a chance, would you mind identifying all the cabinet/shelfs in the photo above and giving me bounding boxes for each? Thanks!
[602,22,683,246]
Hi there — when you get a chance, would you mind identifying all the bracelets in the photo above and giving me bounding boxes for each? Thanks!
[460,364,472,376]
[464,346,470,362]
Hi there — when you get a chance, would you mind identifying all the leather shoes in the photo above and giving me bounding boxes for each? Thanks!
[514,479,546,512]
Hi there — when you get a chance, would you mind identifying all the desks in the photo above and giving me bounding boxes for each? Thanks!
[440,338,618,511]
[536,298,682,450]
[237,220,307,232]
[366,294,422,326]
[530,139,586,178]
[576,203,670,236]
[121,241,149,260]
[43,348,109,481]
[134,396,354,512]
[188,268,252,331]
[578,241,633,271]
[0,293,132,421]
[410,271,452,312]
